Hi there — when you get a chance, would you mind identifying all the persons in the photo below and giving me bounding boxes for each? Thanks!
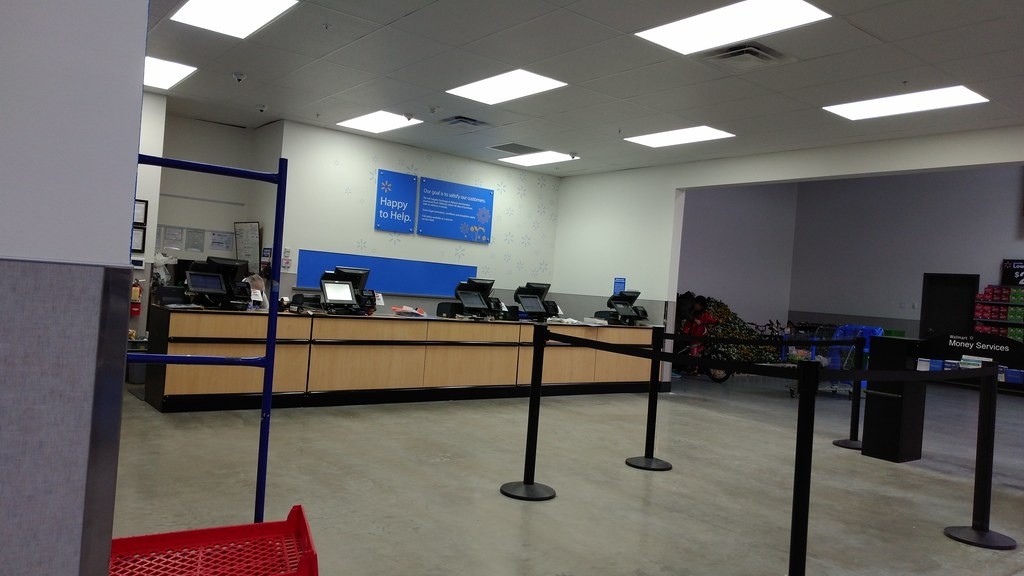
[241,274,269,310]
[682,295,722,377]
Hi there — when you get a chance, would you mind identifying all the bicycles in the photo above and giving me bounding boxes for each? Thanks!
[675,321,732,384]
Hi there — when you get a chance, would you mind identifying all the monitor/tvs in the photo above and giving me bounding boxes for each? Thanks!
[517,283,551,314]
[612,291,640,317]
[458,277,495,309]
[174,256,249,293]
[321,266,370,304]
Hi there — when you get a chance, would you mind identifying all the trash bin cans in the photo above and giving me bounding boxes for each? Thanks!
[127,340,149,384]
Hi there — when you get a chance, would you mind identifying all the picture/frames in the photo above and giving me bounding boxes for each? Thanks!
[132,226,146,253]
[133,199,148,226]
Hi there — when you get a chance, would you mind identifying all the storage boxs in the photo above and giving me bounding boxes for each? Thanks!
[943,360,960,371]
[109,505,319,576]
[929,360,944,371]
[1005,368,1024,384]
[998,365,1008,382]
[916,358,930,371]
[975,285,1024,342]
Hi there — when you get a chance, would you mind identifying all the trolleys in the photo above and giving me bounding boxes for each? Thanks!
[782,325,883,400]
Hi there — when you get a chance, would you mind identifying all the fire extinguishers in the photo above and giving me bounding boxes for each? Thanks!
[130,278,147,315]
